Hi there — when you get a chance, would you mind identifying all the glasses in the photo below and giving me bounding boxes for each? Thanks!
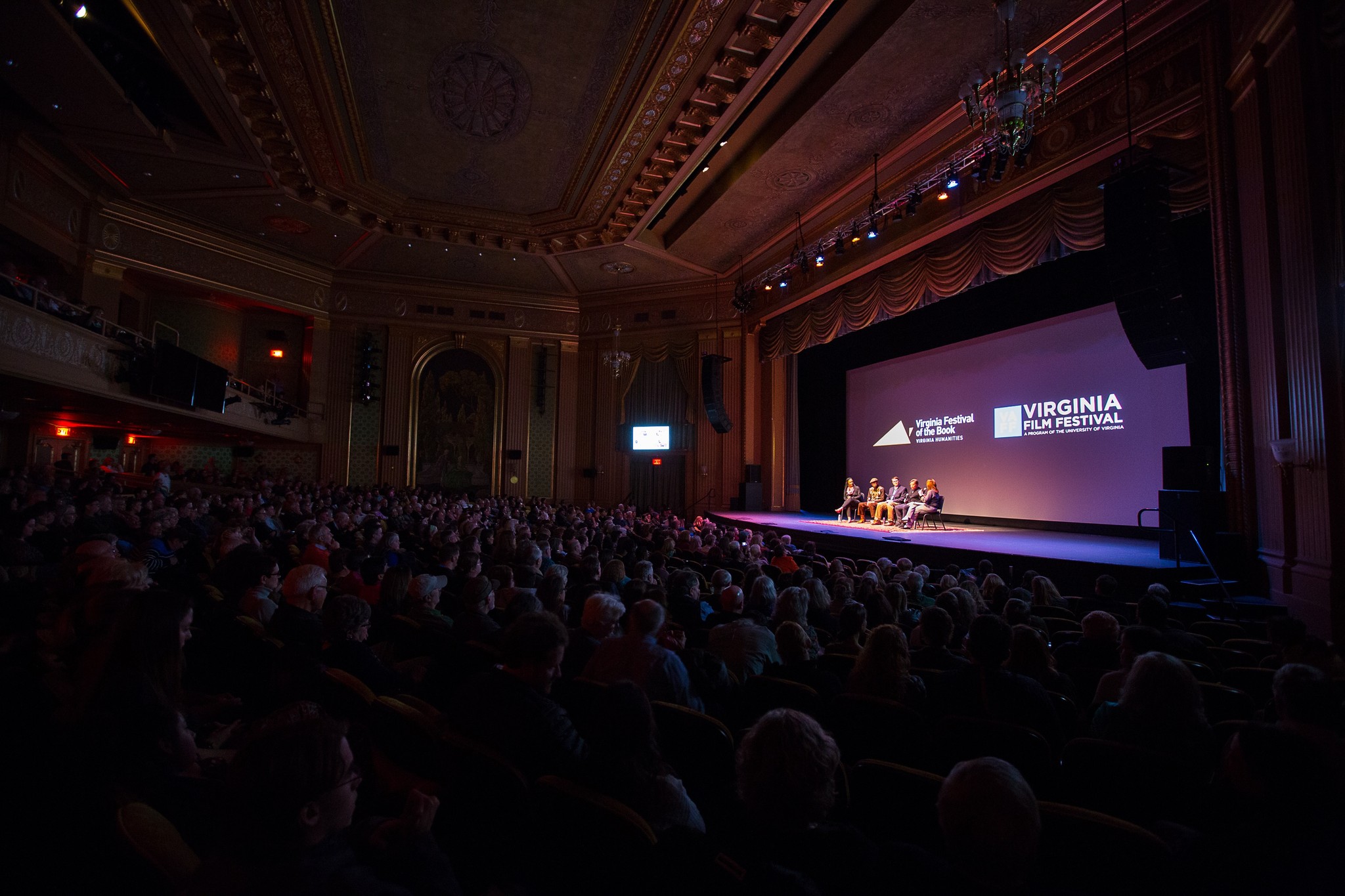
[451,535,457,538]
[578,544,582,546]
[562,587,569,592]
[646,553,650,556]
[433,588,442,591]
[476,561,483,566]
[361,621,370,627]
[68,512,76,515]
[547,548,552,551]
[319,585,331,592]
[163,518,171,522]
[258,513,266,514]
[271,572,281,577]
[513,536,516,538]
[99,549,118,556]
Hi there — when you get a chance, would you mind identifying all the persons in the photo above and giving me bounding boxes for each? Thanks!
[0,273,1345,895]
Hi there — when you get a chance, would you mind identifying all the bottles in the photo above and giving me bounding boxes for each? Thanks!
[914,521,916,529]
[838,514,841,522]
[883,517,885,524]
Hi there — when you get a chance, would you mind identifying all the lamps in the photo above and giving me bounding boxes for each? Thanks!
[778,272,790,288]
[891,202,903,223]
[815,243,825,268]
[507,463,518,484]
[937,182,949,201]
[945,163,960,189]
[969,135,1036,185]
[762,271,777,291]
[1267,438,1316,476]
[700,466,708,478]
[956,0,1065,158]
[728,284,758,316]
[905,184,924,219]
[600,262,636,379]
[850,220,861,243]
[866,203,879,241]
[834,231,846,256]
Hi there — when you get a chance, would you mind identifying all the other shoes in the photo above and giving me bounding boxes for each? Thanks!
[900,525,910,529]
[857,518,866,523]
[898,523,905,528]
[871,520,882,525]
[895,522,901,526]
[846,520,852,523]
[885,522,895,526]
[835,507,843,513]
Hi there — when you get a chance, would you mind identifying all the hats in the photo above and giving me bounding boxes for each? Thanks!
[409,574,448,601]
[870,478,878,483]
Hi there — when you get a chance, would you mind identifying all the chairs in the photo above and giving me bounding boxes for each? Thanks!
[882,507,897,524]
[841,492,865,520]
[0,466,1345,896]
[865,493,888,511]
[914,496,946,530]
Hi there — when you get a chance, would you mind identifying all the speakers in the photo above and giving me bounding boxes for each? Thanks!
[508,450,522,460]
[731,497,744,510]
[1162,445,1201,492]
[1159,490,1205,563]
[702,354,734,432]
[745,465,762,483]
[384,445,400,456]
[739,482,762,512]
[586,468,596,478]
[1104,146,1187,370]
